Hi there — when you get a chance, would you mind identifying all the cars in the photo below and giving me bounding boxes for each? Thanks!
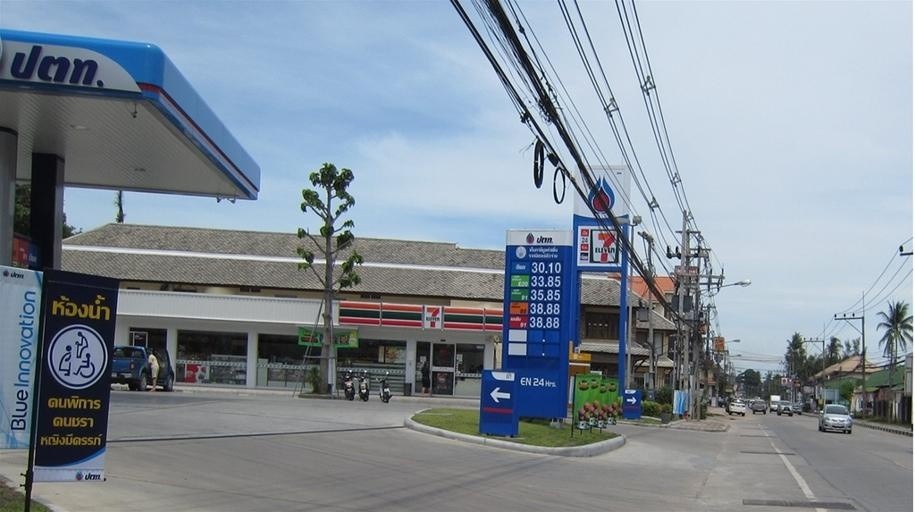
[749,394,802,416]
[819,404,854,434]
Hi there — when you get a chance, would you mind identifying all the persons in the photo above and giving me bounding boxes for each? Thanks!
[420,361,432,398]
[146,350,160,392]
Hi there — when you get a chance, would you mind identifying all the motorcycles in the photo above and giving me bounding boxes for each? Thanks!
[341,369,393,403]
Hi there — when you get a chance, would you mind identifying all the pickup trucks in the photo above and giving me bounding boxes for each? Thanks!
[111,344,175,391]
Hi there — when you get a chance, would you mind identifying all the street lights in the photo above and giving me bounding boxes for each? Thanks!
[693,279,752,416]
[616,215,642,390]
